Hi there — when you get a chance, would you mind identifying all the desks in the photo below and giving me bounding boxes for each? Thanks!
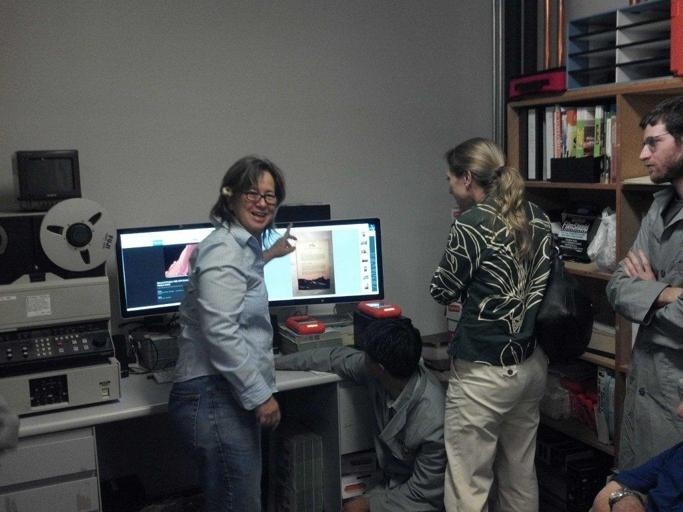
[0,367,377,512]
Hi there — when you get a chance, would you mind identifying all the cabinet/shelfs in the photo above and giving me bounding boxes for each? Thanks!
[507,82,618,458]
[619,76,683,458]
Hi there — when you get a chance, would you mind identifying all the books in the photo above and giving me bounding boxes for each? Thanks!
[519,106,616,184]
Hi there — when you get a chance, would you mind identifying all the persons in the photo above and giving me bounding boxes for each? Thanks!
[430,135,556,512]
[585,400,682,512]
[271,314,447,512]
[604,94,683,471]
[167,151,299,511]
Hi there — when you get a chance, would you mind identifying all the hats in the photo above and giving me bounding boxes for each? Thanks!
[355,316,424,377]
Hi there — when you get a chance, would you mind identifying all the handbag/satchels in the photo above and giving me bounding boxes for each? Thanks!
[533,258,596,369]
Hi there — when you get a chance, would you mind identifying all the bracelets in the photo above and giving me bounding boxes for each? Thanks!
[608,488,646,512]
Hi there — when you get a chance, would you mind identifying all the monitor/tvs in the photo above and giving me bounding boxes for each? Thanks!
[115,222,218,331]
[11,149,82,213]
[262,218,384,326]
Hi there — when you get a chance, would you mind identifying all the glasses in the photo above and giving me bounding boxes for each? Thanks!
[642,131,670,153]
[239,190,277,206]
